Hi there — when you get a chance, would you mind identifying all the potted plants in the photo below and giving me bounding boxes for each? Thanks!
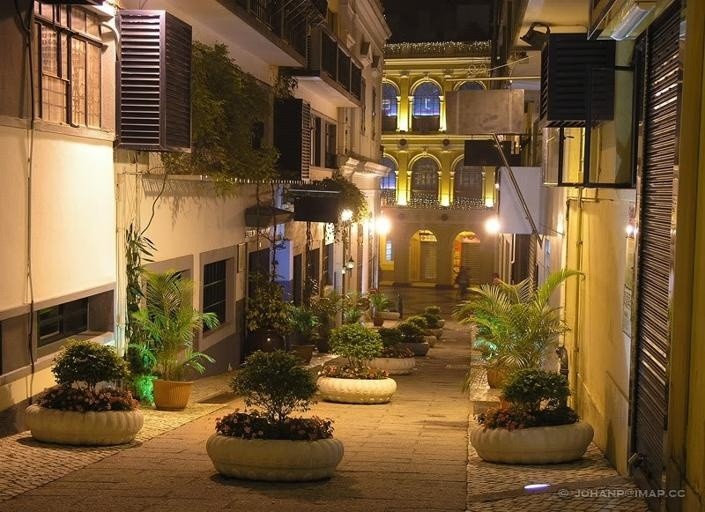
[396,321,429,357]
[450,268,588,410]
[27,339,144,446]
[371,291,392,327]
[365,327,416,374]
[472,369,594,465]
[289,306,321,363]
[205,348,345,482]
[131,270,221,412]
[316,322,397,406]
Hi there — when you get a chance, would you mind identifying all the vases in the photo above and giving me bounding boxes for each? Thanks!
[405,317,437,347]
[421,314,443,339]
[425,306,445,328]
[486,363,510,389]
[316,337,330,353]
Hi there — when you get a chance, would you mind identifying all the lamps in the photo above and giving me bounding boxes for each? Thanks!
[520,21,551,50]
[622,219,635,243]
[348,255,354,269]
[342,265,346,275]
